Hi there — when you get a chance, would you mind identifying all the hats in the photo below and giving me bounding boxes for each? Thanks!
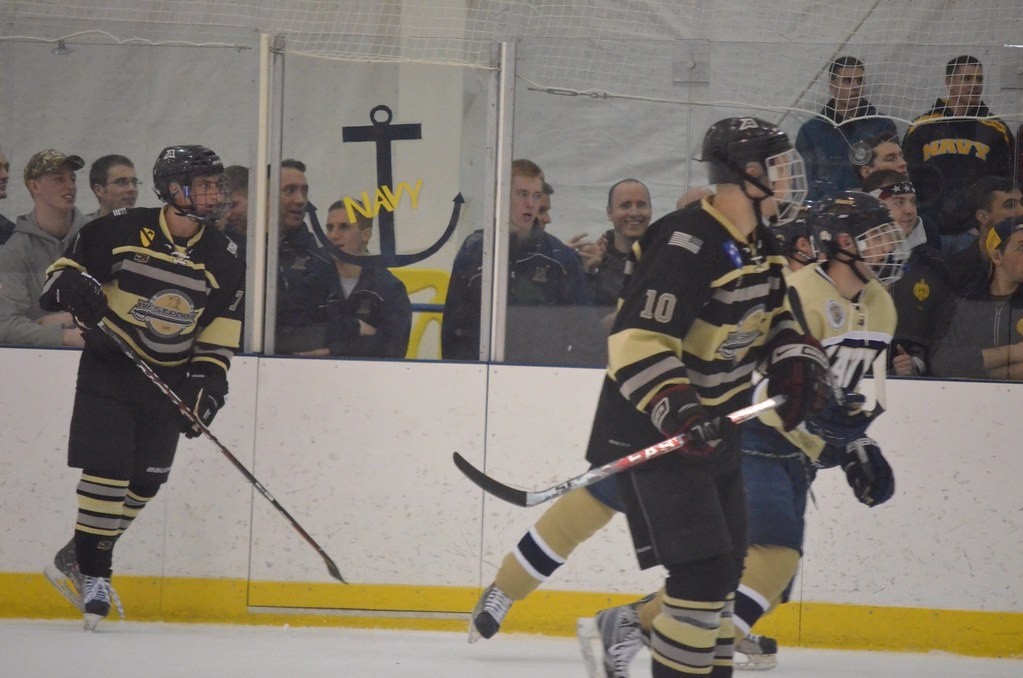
[23,148,85,182]
[986,215,1023,283]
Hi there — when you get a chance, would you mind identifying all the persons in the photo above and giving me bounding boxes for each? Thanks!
[603,116,829,678]
[441,159,608,368]
[209,159,319,358]
[939,176,1023,379]
[583,179,652,307]
[846,132,943,379]
[676,183,714,209]
[293,198,412,358]
[471,190,912,678]
[0,148,139,347]
[796,56,900,215]
[902,55,1016,207]
[39,144,245,618]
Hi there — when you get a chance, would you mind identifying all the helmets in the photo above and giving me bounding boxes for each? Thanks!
[701,119,810,226]
[811,190,910,287]
[153,144,233,225]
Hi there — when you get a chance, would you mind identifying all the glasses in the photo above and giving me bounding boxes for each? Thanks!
[101,177,144,188]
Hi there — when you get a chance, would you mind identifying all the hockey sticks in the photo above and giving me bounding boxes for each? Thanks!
[453,395,789,507]
[96,323,348,583]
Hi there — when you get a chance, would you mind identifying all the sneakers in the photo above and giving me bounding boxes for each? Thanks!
[44,530,85,613]
[81,570,126,631]
[468,583,514,644]
[732,632,778,670]
[575,596,653,678]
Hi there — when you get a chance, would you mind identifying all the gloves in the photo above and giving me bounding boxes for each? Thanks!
[841,436,894,507]
[648,383,742,469]
[175,374,228,439]
[767,332,829,433]
[49,270,109,332]
